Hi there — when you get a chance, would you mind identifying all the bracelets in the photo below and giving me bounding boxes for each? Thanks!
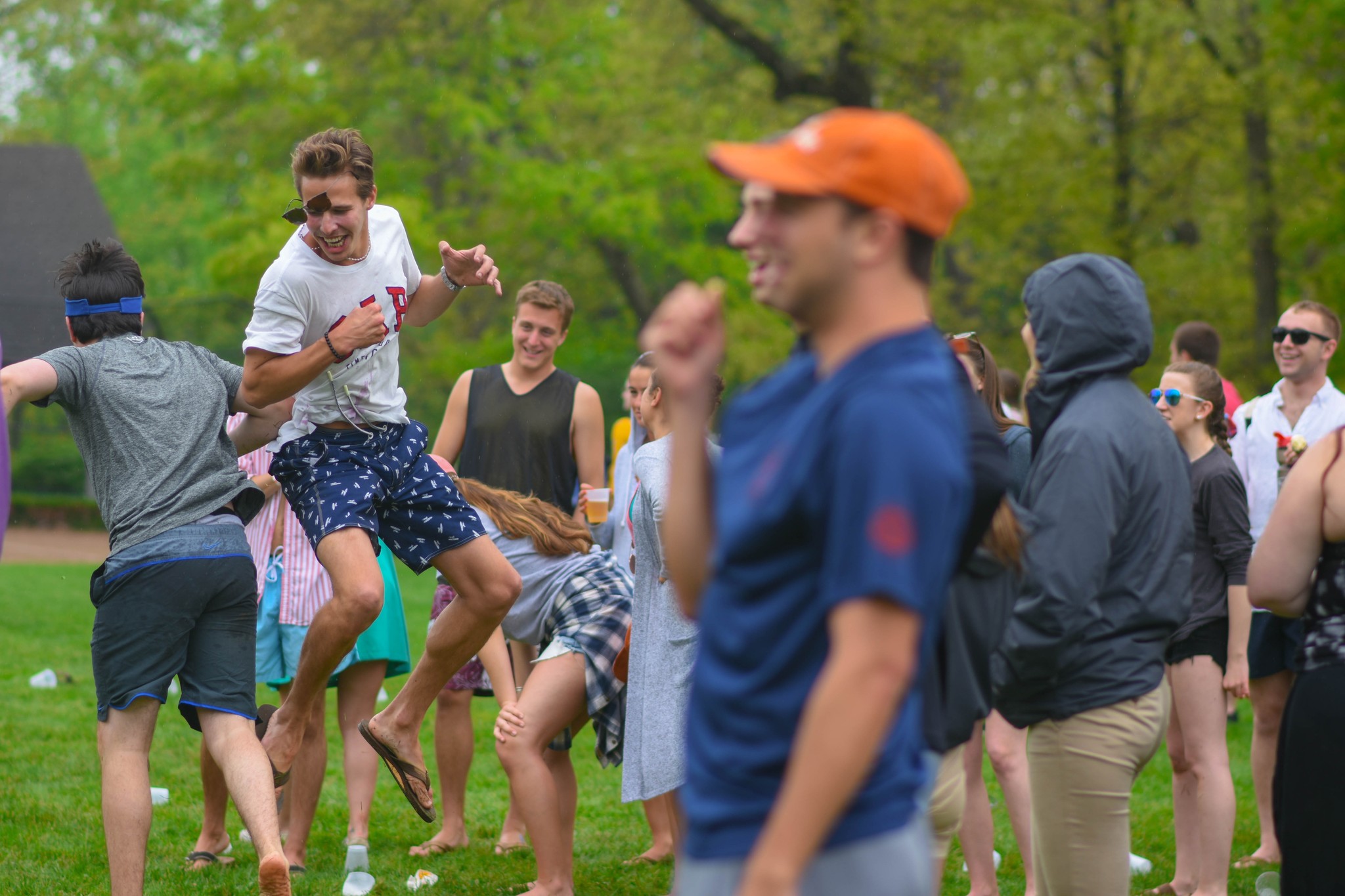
[324,333,347,359]
[441,266,466,291]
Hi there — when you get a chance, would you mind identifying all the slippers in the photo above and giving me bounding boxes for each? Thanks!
[624,856,657,865]
[256,704,292,815]
[183,851,235,871]
[288,865,304,877]
[358,720,437,824]
[409,841,469,860]
[495,841,529,857]
[1232,856,1270,869]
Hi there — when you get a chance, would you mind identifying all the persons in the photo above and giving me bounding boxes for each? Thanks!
[185,105,1345,896]
[1,240,291,896]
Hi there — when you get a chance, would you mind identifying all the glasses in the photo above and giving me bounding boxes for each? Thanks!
[1271,327,1331,345]
[282,171,359,225]
[948,331,980,346]
[1150,387,1208,406]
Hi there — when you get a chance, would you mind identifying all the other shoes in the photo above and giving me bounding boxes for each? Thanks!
[1142,883,1193,896]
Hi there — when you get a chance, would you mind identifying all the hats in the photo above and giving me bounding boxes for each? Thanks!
[709,108,968,236]
[426,453,459,479]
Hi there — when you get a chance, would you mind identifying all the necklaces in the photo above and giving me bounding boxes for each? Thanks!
[298,224,370,261]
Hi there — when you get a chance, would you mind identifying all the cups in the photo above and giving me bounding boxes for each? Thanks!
[1254,871,1281,896]
[344,845,369,871]
[150,787,169,805]
[342,871,375,896]
[1130,852,1152,874]
[963,850,1001,872]
[584,488,609,522]
[29,668,58,690]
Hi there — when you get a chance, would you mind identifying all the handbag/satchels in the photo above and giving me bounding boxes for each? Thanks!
[920,542,1018,756]
